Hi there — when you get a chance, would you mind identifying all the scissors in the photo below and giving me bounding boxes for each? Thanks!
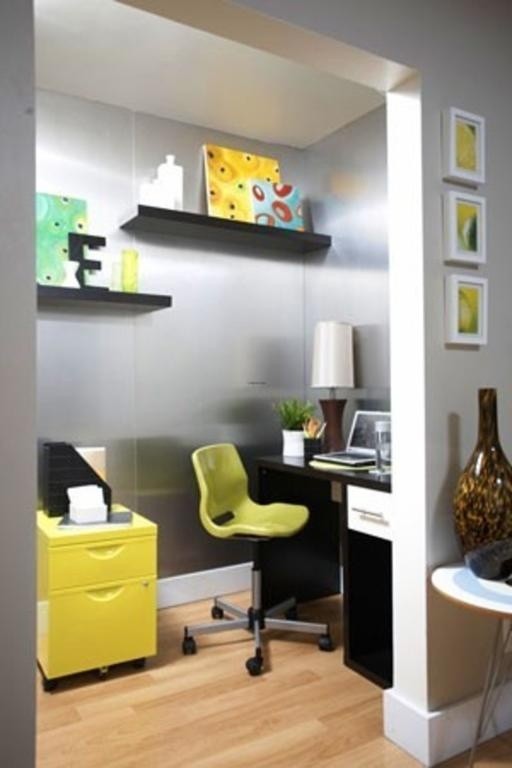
[305,417,321,439]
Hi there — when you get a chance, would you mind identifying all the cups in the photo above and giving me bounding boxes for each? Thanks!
[123,250,139,292]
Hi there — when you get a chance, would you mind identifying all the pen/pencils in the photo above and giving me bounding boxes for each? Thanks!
[302,422,327,440]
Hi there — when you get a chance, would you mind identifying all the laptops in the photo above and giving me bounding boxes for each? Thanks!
[313,410,391,466]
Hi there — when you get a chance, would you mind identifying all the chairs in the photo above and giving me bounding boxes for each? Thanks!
[183,442,335,676]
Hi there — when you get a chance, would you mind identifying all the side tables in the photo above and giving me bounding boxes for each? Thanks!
[431,561,512,768]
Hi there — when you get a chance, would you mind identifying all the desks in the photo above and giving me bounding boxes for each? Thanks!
[244,448,394,690]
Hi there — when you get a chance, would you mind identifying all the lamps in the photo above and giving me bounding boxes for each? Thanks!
[309,320,355,452]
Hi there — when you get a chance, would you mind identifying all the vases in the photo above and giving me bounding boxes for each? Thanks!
[454,387,512,581]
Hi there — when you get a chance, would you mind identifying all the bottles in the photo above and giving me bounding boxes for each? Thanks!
[375,419,392,473]
[158,154,184,210]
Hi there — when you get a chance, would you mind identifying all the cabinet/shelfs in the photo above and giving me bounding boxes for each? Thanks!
[34,501,159,692]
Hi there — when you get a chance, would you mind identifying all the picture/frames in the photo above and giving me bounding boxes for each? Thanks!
[441,105,485,188]
[443,190,486,265]
[445,273,490,346]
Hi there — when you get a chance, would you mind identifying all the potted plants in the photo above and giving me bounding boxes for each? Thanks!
[280,399,315,457]
